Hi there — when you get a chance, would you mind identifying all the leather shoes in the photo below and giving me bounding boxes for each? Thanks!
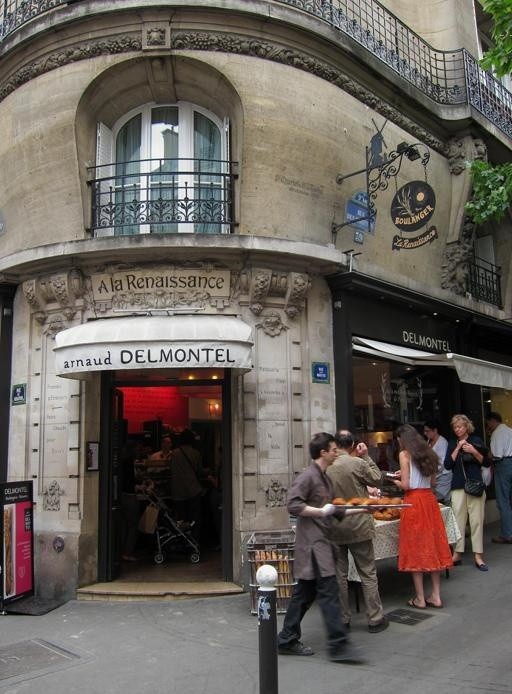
[453,560,462,566]
[475,561,488,571]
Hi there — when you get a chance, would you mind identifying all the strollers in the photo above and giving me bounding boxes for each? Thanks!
[142,487,202,564]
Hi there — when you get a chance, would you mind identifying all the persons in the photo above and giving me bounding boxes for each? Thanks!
[424,422,454,506]
[488,412,512,543]
[445,413,491,571]
[327,430,388,633]
[118,427,223,562]
[277,432,368,664]
[384,423,454,609]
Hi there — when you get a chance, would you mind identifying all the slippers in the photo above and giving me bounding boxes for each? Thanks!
[425,601,443,608]
[408,598,427,609]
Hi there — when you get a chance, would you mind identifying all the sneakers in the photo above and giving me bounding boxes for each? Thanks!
[368,617,389,633]
[278,641,314,655]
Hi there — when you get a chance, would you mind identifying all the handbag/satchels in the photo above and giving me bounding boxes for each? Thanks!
[464,477,485,497]
